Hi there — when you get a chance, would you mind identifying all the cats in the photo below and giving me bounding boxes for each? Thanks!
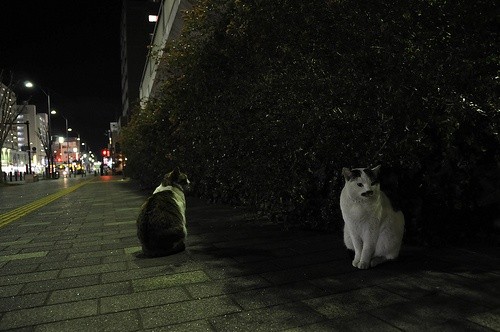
[340,164,405,270]
[136,167,191,258]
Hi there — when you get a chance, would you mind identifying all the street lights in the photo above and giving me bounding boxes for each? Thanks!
[49,109,72,179]
[81,142,98,177]
[24,80,54,181]
[66,126,82,179]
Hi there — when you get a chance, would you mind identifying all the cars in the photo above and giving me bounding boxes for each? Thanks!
[54,163,69,177]
